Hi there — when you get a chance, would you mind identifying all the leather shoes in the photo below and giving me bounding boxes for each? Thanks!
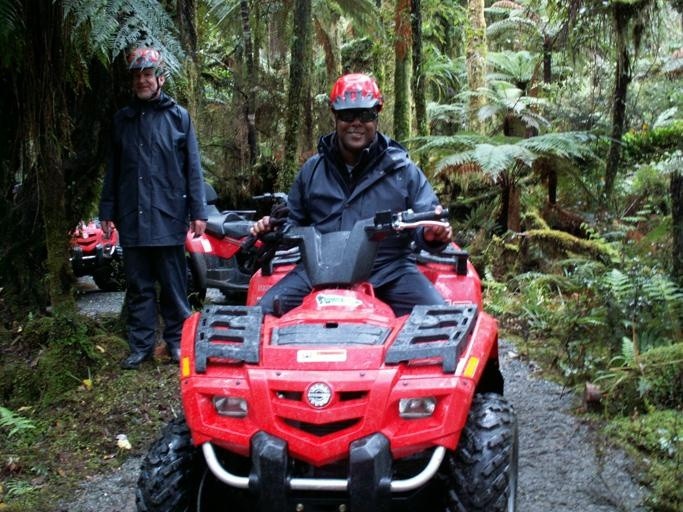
[171,348,181,361]
[120,351,152,368]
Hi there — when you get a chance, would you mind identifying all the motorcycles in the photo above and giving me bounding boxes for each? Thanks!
[66,182,290,310]
[135,208,519,511]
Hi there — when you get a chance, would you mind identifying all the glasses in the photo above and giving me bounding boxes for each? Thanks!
[338,110,378,122]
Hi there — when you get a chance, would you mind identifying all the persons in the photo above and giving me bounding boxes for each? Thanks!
[99,46,207,367]
[250,74,453,320]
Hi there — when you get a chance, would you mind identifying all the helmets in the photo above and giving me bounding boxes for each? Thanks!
[127,49,160,70]
[329,74,383,112]
